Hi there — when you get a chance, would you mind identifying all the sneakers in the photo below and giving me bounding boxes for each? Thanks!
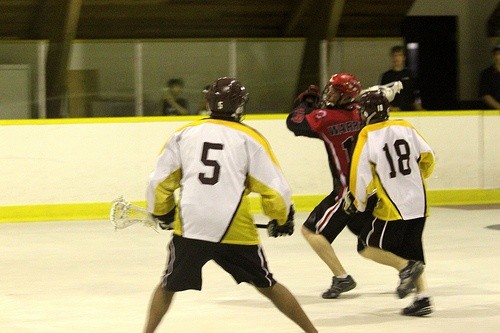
[397,261,424,299]
[322,275,356,298]
[403,297,432,316]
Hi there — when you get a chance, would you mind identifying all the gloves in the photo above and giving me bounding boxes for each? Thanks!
[267,205,295,238]
[339,192,377,216]
[294,84,320,108]
[152,205,176,230]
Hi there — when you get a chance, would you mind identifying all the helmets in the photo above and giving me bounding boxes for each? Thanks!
[208,77,246,116]
[358,92,390,119]
[325,73,361,109]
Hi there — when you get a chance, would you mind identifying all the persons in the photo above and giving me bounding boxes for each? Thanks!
[141,78,318,333]
[159,78,190,115]
[378,44,424,111]
[343,86,434,316]
[477,45,500,112]
[282,73,376,300]
